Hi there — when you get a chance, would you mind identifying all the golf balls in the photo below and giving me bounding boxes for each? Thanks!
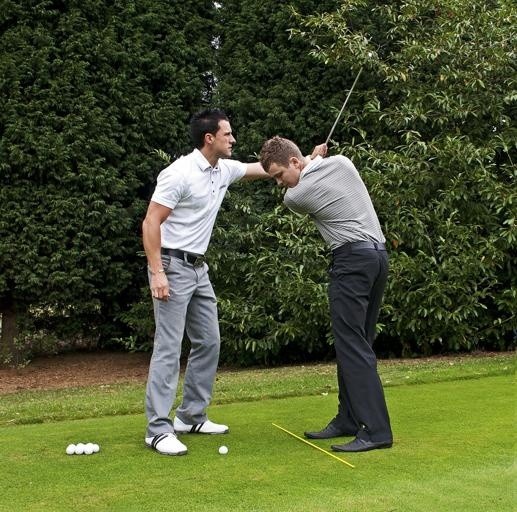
[66,443,99,455]
[219,446,227,455]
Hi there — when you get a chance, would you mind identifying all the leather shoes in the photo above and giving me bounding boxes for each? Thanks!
[304,424,352,438]
[331,437,394,452]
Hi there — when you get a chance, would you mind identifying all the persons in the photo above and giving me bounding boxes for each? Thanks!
[141,106,328,456]
[259,135,395,452]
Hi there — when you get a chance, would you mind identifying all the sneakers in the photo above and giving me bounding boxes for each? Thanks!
[174,415,229,436]
[145,433,188,455]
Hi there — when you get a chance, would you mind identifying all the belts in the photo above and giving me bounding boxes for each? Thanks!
[333,241,387,253]
[161,247,205,267]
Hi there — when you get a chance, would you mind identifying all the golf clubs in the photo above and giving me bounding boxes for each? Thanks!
[326,52,378,144]
